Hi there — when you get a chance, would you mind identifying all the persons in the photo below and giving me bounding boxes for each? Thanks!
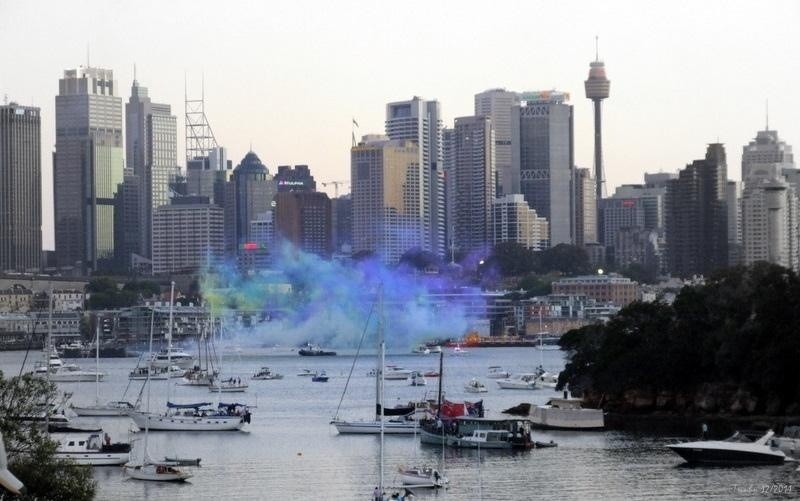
[701,422,708,441]
[372,486,415,501]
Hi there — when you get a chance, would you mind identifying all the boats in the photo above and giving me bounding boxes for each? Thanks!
[294,282,632,501]
[665,425,800,465]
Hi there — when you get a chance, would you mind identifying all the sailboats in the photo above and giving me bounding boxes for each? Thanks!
[0,281,283,481]
